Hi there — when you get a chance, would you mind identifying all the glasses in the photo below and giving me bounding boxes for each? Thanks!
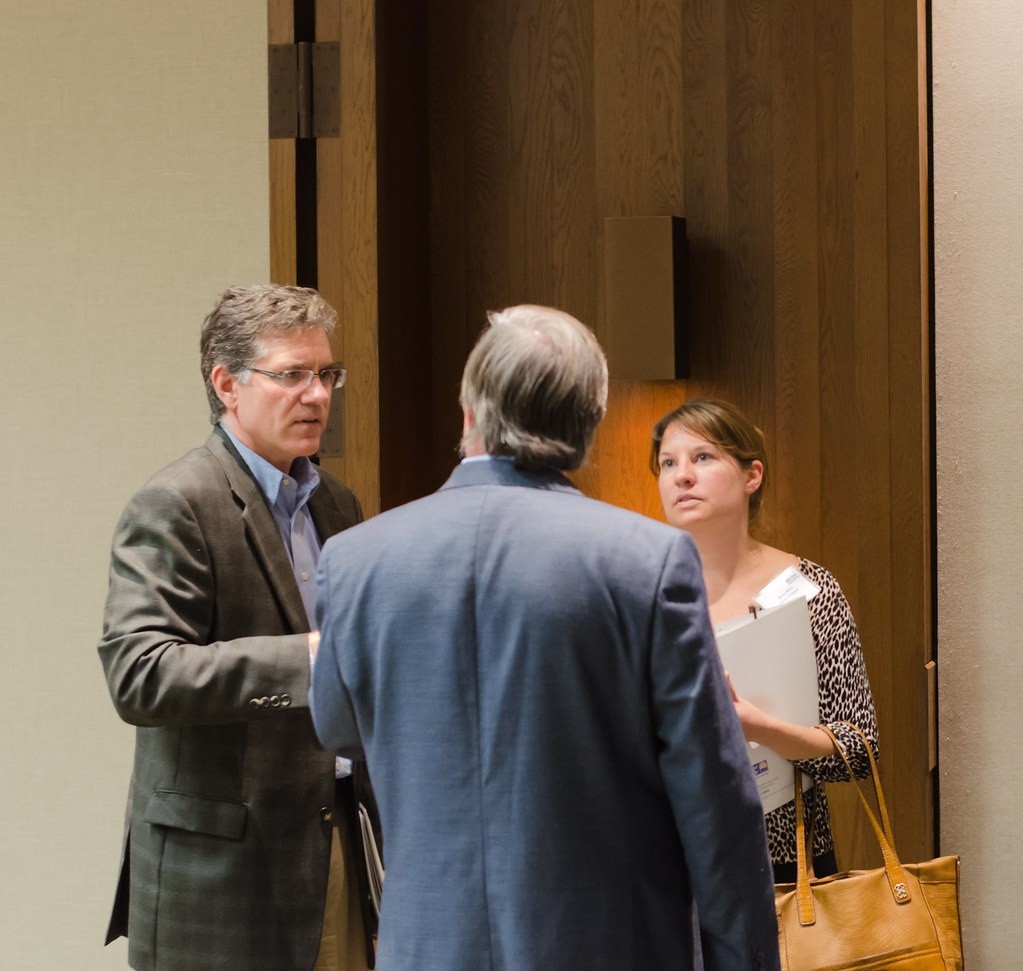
[227,362,349,391]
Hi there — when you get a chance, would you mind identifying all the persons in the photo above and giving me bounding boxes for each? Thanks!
[308,304,782,971]
[649,397,881,886]
[97,283,364,971]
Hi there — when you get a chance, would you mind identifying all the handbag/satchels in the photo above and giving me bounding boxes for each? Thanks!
[774,722,964,971]
